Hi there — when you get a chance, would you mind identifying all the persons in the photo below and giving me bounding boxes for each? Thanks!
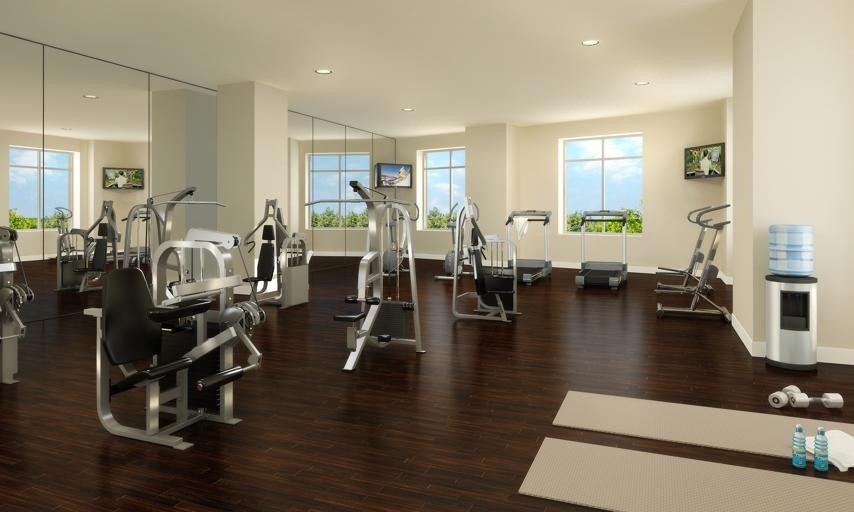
[114,171,128,187]
[701,149,713,175]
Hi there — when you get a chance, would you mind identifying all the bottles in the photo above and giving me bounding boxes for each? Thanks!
[791,423,831,473]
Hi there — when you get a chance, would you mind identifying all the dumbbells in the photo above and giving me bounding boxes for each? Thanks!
[769,385,845,409]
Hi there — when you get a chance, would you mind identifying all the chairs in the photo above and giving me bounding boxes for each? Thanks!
[244,224,276,304]
[102,267,193,392]
[71,223,107,292]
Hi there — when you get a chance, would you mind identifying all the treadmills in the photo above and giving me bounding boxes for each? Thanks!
[495,208,552,286]
[574,211,628,290]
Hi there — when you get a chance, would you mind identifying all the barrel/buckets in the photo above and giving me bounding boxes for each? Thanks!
[768,224,815,277]
